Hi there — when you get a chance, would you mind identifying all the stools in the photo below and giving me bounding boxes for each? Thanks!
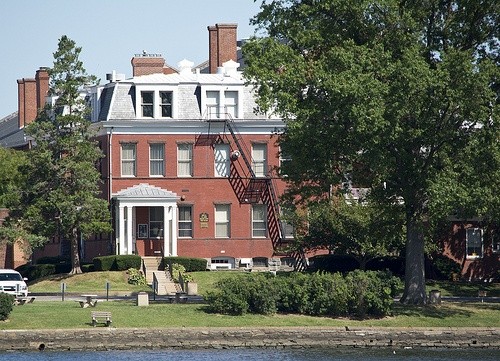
[166,296,188,304]
[76,299,99,308]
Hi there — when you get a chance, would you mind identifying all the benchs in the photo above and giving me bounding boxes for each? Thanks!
[90,310,114,327]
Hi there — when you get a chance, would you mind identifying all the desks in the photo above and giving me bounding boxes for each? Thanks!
[81,294,97,306]
[171,291,187,304]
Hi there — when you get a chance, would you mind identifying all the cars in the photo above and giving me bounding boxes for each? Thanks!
[0,269,28,296]
